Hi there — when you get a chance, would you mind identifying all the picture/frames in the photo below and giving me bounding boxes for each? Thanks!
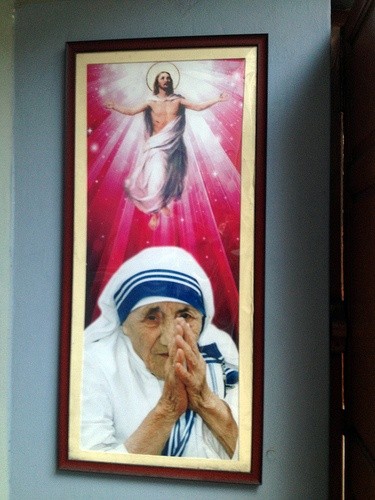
[53,31,271,494]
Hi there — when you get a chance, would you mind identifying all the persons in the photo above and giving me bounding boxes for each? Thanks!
[104,71,229,232]
[82,244,239,460]
